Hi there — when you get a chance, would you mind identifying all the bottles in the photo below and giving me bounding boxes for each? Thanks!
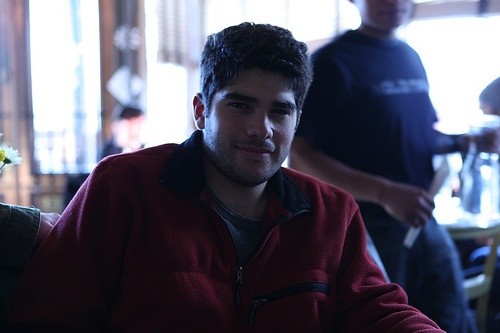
[461,142,481,213]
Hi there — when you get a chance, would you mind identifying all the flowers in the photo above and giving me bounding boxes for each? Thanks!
[0,144,23,170]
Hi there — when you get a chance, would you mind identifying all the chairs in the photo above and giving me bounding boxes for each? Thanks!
[441,225,500,333]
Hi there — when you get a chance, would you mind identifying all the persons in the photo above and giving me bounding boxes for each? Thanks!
[0,203,62,333]
[11,23,448,333]
[97,104,147,164]
[287,0,500,333]
